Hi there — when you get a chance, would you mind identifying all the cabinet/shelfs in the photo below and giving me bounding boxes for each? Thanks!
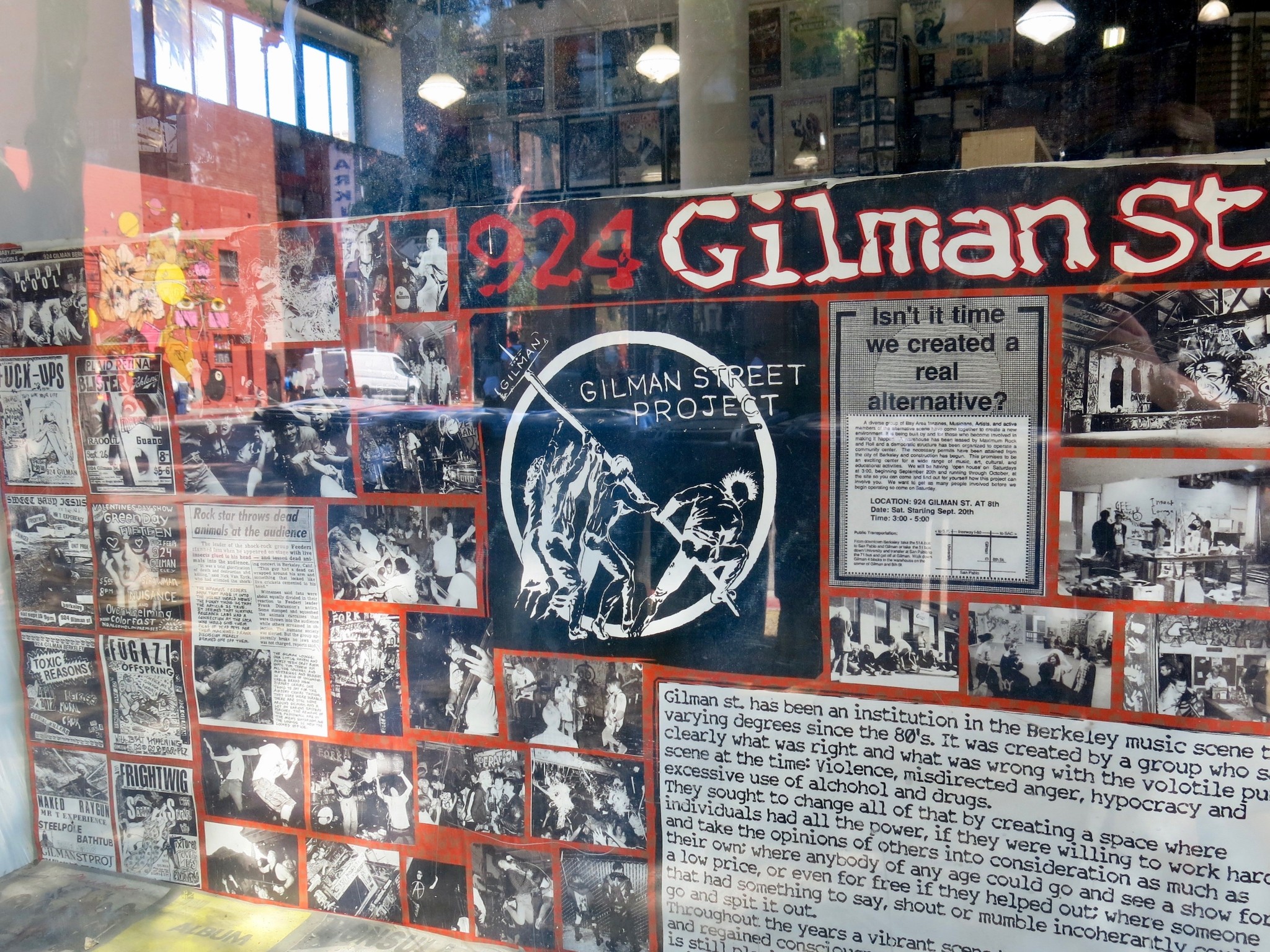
[134,77,403,233]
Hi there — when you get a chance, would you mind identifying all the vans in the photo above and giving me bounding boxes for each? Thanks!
[300,349,423,404]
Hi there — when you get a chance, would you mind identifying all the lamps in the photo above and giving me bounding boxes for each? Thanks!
[1197,0,1230,23]
[417,0,466,110]
[635,0,680,84]
[1015,0,1076,46]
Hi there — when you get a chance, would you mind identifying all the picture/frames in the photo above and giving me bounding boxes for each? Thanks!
[665,104,680,184]
[551,28,600,113]
[747,0,1012,179]
[602,21,674,106]
[456,45,501,123]
[613,106,665,188]
[564,111,613,193]
[502,32,549,117]
[515,116,563,197]
[461,118,516,203]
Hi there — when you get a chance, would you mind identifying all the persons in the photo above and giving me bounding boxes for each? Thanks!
[1041,80,1269,441]
[0,222,1270,952]
